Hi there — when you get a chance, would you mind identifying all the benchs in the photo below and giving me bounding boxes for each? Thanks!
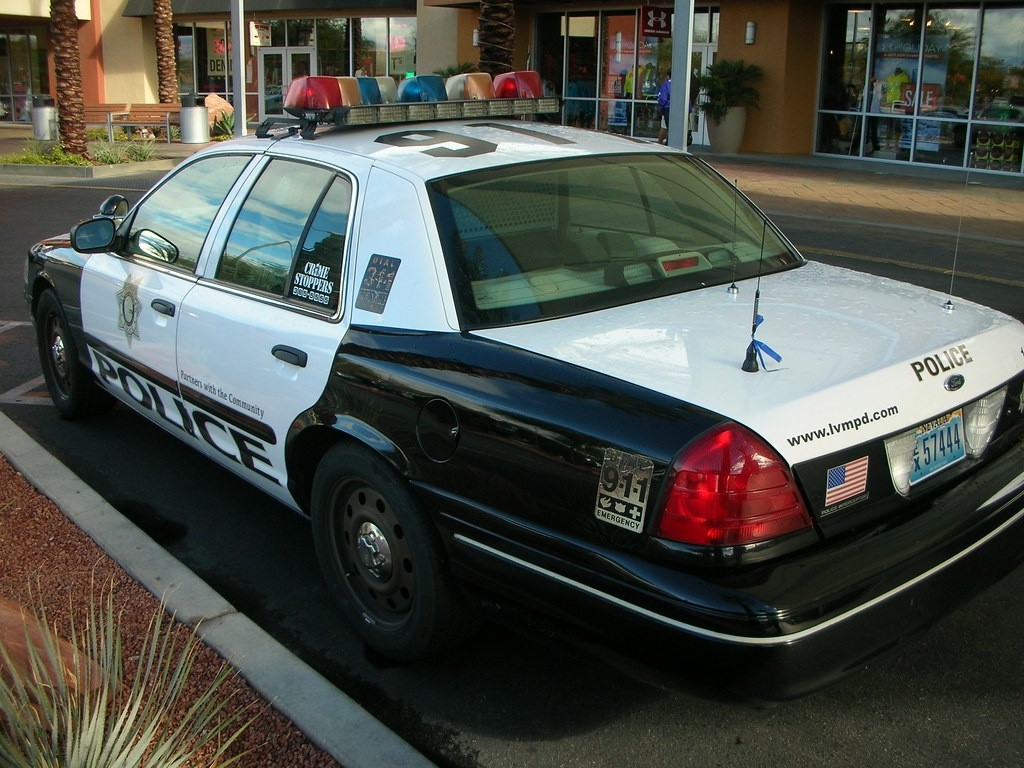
[105,103,182,145]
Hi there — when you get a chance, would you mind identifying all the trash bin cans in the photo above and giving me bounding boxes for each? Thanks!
[179,90,211,145]
[30,93,59,142]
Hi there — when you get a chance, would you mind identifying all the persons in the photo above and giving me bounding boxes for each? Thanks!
[624,62,657,137]
[355,59,368,77]
[885,67,909,153]
[866,77,884,151]
[657,67,700,146]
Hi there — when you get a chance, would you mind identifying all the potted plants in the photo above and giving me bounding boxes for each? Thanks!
[694,59,764,156]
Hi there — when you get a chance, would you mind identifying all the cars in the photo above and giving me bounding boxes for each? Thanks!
[879,94,1024,164]
[21,71,1024,709]
